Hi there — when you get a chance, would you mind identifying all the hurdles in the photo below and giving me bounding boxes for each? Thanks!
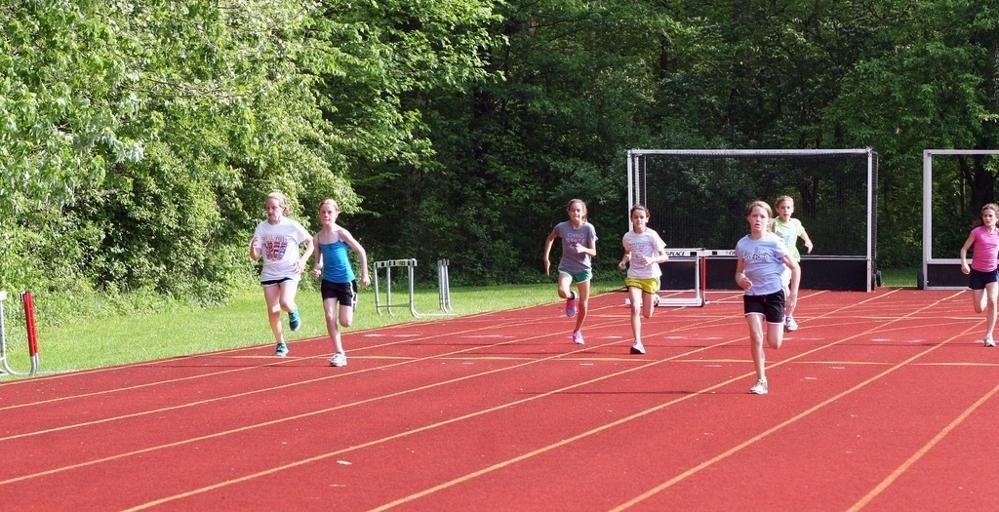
[373,258,461,318]
[625,246,737,307]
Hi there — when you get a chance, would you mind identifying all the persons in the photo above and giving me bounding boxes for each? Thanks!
[767,195,813,332]
[618,204,670,356]
[736,201,797,396]
[312,200,370,367]
[249,192,313,357]
[961,202,999,348]
[544,199,601,345]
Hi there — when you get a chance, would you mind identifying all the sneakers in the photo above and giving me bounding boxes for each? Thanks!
[750,378,767,394]
[786,317,798,331]
[566,292,578,317]
[574,330,585,344]
[655,294,659,307]
[289,308,300,331]
[984,338,996,347]
[329,353,347,367]
[630,345,645,354]
[275,344,288,356]
